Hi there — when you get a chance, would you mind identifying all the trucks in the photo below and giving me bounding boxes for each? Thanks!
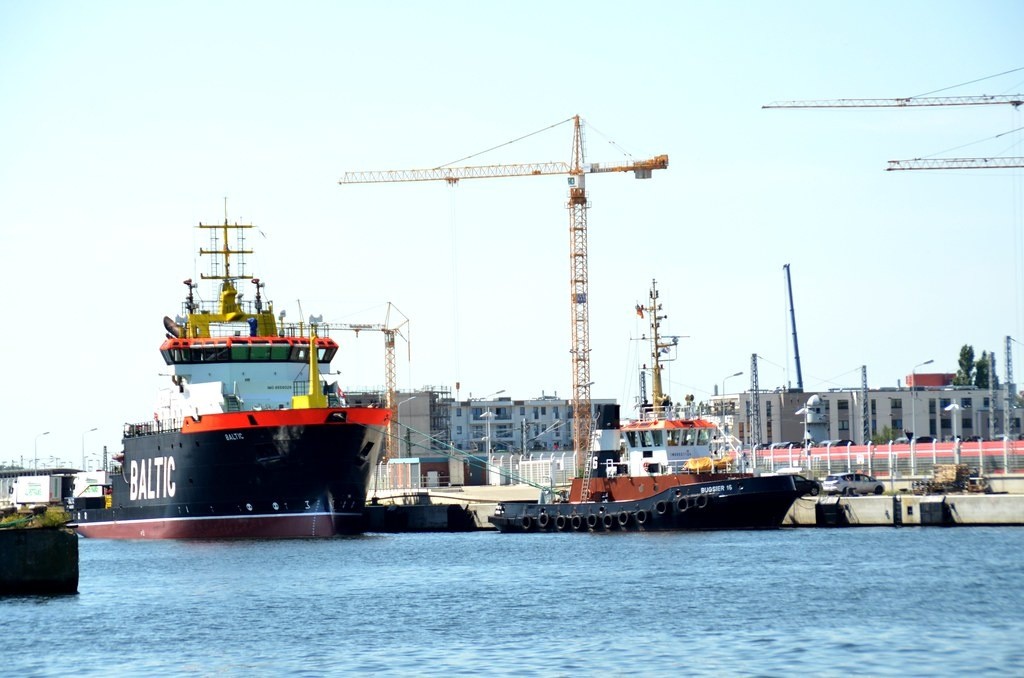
[913,464,970,496]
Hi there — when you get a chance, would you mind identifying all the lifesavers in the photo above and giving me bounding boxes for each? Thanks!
[643,462,649,471]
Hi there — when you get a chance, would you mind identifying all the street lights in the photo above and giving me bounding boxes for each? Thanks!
[943,398,965,464]
[911,360,935,473]
[82,428,98,472]
[33,431,51,477]
[721,372,743,431]
[794,403,817,470]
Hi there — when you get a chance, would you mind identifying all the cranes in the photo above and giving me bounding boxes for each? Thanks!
[276,299,410,461]
[336,112,670,477]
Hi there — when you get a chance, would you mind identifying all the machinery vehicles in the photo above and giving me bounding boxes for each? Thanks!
[966,477,994,494]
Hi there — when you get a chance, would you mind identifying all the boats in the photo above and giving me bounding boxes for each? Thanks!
[0,516,80,595]
[487,416,815,530]
[362,487,478,534]
[822,474,885,496]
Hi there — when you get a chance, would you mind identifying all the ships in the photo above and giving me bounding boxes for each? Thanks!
[67,206,395,539]
